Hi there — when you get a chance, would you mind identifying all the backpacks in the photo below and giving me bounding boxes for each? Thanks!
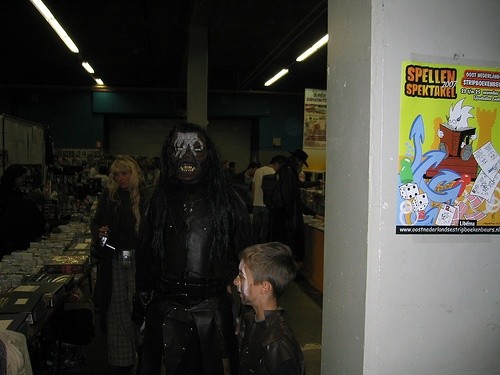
[260,174,281,212]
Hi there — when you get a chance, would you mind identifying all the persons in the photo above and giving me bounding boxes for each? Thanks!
[233,242,307,375]
[226,161,260,209]
[134,122,266,375]
[90,155,155,375]
[0,163,48,262]
[269,149,309,278]
[250,154,286,244]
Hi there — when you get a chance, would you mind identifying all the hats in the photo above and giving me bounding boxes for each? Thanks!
[288,148,308,168]
